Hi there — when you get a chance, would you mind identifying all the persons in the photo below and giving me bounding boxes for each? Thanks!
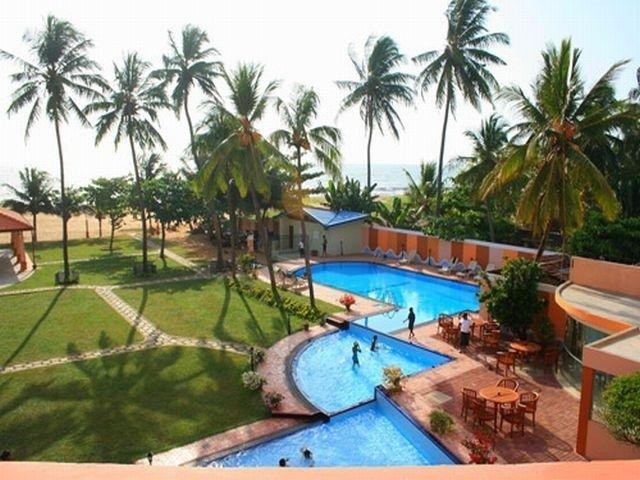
[298,239,305,259]
[458,311,477,353]
[278,458,287,466]
[402,306,416,339]
[351,339,362,370]
[369,334,379,354]
[301,446,314,462]
[321,234,328,259]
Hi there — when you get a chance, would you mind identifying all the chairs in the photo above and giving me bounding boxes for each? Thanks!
[438,256,496,281]
[437,314,560,438]
